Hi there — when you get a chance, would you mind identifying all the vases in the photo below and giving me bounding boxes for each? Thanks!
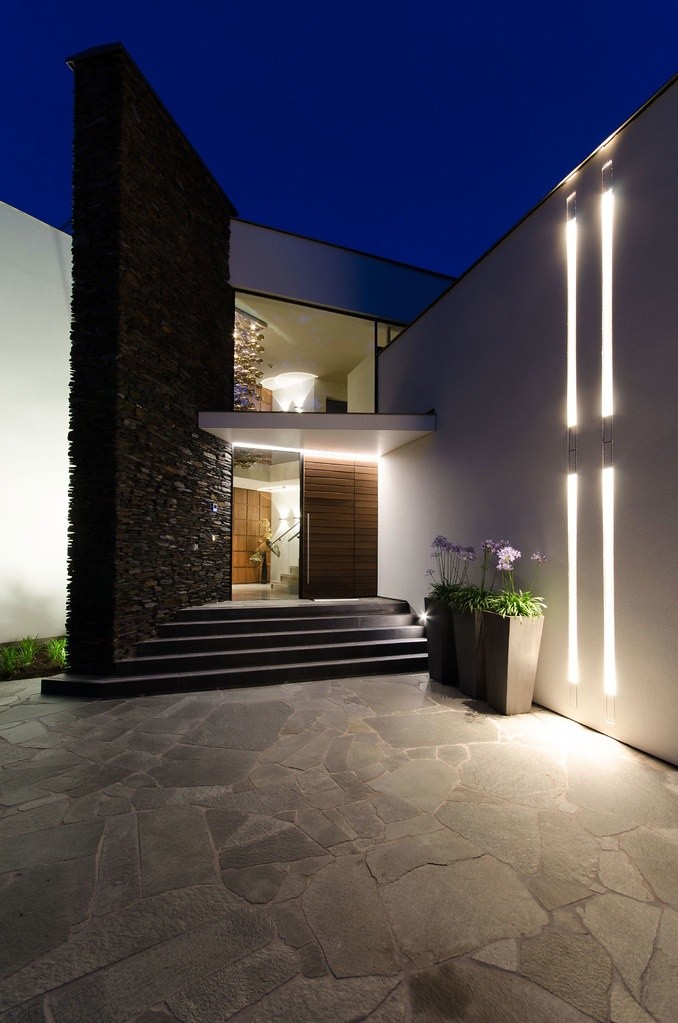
[448,602,490,698]
[483,611,546,716]
[424,596,458,685]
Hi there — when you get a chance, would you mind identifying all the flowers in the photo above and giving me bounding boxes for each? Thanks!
[423,535,550,624]
